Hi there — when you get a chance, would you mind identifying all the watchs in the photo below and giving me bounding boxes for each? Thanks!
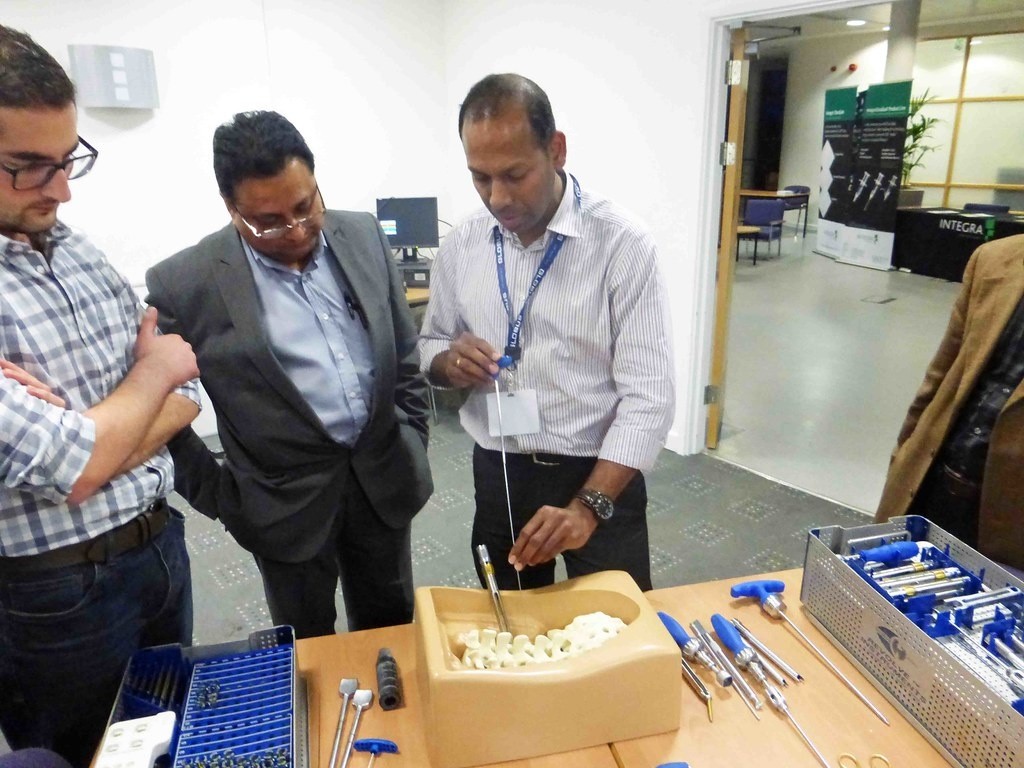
[575,487,615,521]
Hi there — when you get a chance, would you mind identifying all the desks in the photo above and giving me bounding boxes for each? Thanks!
[91,567,949,768]
[737,225,761,267]
[890,206,1024,283]
[741,190,810,244]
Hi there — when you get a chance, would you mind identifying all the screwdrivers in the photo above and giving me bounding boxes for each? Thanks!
[705,613,830,768]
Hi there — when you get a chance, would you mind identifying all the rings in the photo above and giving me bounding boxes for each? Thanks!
[456,358,462,368]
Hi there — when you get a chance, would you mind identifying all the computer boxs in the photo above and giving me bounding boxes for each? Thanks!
[395,259,434,287]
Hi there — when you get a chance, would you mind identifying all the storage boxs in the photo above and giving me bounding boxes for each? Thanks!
[798,513,1024,767]
[418,569,681,768]
[98,623,314,768]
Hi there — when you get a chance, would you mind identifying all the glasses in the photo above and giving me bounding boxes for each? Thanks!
[0,133,98,190]
[224,181,328,240]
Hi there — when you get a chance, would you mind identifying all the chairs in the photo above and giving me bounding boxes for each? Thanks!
[778,186,811,239]
[737,200,785,265]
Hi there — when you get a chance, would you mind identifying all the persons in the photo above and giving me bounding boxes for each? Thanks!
[0,28,205,762]
[145,103,435,634]
[414,74,683,593]
[872,231,1024,569]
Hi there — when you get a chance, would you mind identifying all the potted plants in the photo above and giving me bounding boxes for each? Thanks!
[899,93,940,208]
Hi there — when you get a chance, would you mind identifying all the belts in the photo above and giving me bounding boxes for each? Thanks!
[0,498,170,573]
[932,460,984,505]
[532,451,600,465]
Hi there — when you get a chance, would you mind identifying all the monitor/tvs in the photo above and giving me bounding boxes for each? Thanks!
[378,197,439,266]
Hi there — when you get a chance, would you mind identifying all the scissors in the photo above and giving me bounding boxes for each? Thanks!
[838,752,895,768]
